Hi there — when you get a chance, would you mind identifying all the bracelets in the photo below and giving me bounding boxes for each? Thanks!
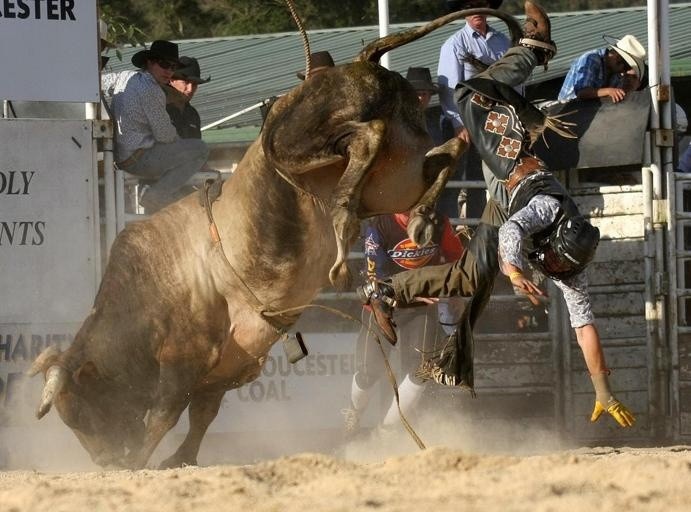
[509,272,522,281]
[587,370,610,376]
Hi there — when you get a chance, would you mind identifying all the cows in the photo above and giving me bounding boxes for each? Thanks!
[24,7,525,471]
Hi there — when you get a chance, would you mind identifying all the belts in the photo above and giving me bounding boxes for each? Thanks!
[110,148,144,171]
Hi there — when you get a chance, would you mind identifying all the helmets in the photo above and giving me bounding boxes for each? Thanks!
[528,215,600,282]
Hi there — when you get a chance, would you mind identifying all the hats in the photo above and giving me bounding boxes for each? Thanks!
[406,67,440,95]
[298,51,335,81]
[602,35,647,83]
[172,56,211,85]
[131,39,188,70]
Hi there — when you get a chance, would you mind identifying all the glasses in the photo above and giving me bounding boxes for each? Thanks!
[156,60,177,74]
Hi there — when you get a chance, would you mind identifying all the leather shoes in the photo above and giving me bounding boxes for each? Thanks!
[519,1,557,65]
[356,282,398,347]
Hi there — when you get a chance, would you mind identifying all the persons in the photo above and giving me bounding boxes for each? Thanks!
[356,0,636,428]
[557,34,690,186]
[436,0,522,236]
[100,39,210,216]
[164,55,210,139]
[339,208,465,456]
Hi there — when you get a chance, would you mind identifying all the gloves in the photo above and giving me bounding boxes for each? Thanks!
[508,272,544,307]
[590,370,636,429]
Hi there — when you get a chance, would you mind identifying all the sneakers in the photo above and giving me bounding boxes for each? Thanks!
[343,402,400,442]
[139,187,171,211]
